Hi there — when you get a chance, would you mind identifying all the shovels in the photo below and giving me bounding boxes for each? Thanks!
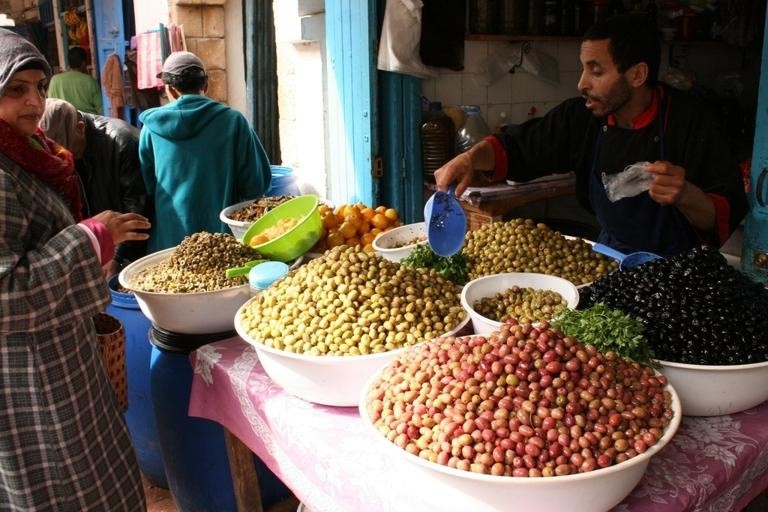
[225,259,271,281]
[591,241,667,276]
[427,182,468,257]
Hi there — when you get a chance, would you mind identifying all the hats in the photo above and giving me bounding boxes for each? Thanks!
[1,26,53,95]
[156,50,207,80]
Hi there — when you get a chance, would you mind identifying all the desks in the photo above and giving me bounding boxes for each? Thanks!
[188,338,768,512]
[423,174,576,230]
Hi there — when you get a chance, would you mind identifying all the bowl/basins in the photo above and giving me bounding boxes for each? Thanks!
[240,195,322,263]
[120,247,304,339]
[235,285,472,407]
[575,236,622,290]
[360,336,683,509]
[371,221,431,262]
[653,358,768,415]
[219,198,334,248]
[462,273,579,338]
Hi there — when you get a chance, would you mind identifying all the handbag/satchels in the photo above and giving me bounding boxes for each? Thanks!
[93,309,128,413]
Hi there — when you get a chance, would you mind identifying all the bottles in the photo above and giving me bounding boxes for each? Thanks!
[496,105,537,131]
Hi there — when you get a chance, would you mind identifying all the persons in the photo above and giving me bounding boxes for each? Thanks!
[433,16,749,260]
[136,52,271,249]
[36,98,153,277]
[46,45,104,117]
[1,27,151,511]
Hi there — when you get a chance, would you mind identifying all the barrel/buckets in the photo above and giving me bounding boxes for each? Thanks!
[265,167,301,198]
[146,322,294,512]
[457,105,494,156]
[494,113,512,135]
[420,96,457,184]
[108,273,169,493]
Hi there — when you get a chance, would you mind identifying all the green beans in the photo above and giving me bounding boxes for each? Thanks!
[240,244,468,356]
[463,216,621,285]
[474,285,568,318]
[366,317,674,476]
[578,245,767,364]
